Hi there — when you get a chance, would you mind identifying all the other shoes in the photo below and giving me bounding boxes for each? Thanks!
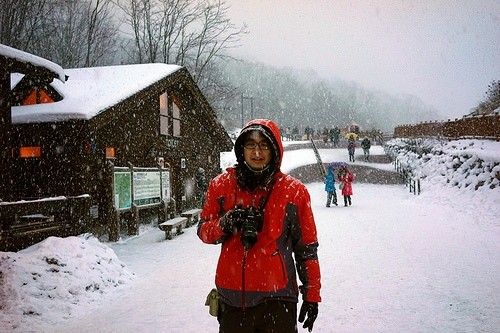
[349,201,351,205]
[344,204,348,207]
[326,205,331,207]
[333,202,338,206]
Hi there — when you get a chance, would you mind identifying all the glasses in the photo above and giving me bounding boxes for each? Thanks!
[242,138,271,150]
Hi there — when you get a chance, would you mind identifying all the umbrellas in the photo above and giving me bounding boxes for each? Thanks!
[345,133,356,139]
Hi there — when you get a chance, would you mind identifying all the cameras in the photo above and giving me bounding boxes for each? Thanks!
[232,207,263,248]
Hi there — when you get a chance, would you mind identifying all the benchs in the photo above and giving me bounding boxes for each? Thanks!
[180,208,203,225]
[159,216,188,240]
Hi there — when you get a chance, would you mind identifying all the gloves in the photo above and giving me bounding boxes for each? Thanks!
[221,202,246,235]
[299,300,318,332]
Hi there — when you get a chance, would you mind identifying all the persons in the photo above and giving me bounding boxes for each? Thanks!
[378,129,385,145]
[324,165,338,207]
[361,136,371,162]
[197,118,322,333]
[350,125,360,140]
[348,135,355,143]
[317,128,321,139]
[348,141,356,162]
[371,127,377,141]
[322,126,341,148]
[279,126,285,141]
[286,126,299,141]
[337,163,354,207]
[304,125,315,139]
